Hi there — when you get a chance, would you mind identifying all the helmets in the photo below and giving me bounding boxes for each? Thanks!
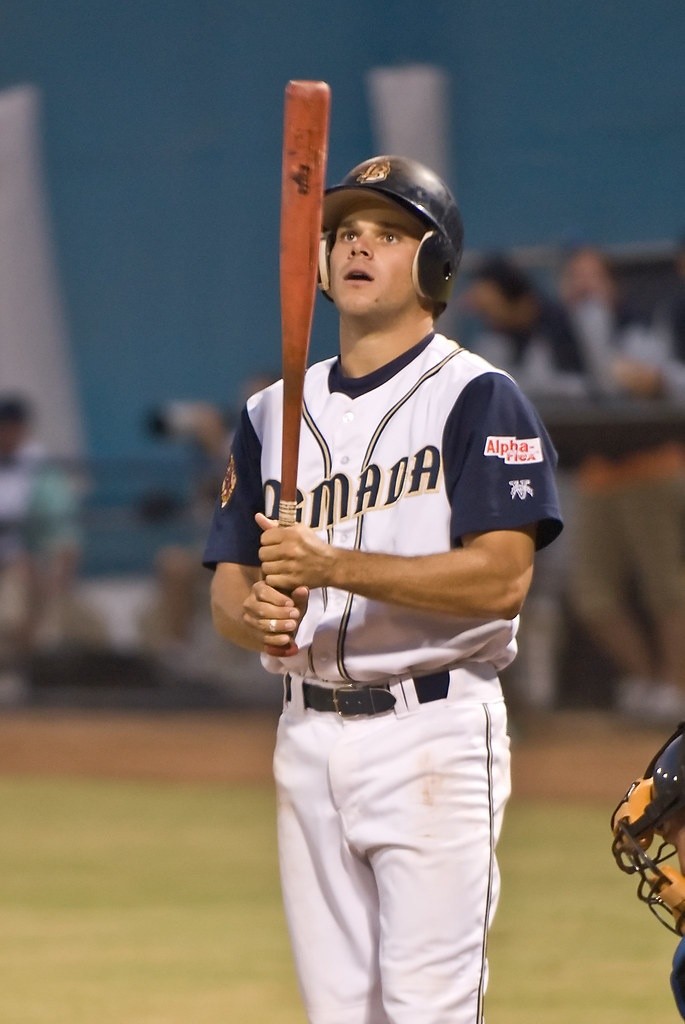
[314,156,465,318]
[643,728,685,838]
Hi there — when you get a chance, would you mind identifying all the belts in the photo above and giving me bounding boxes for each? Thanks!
[284,669,452,717]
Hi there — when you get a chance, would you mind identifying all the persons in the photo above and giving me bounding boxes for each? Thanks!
[0,389,86,708]
[203,154,566,1023]
[143,372,268,655]
[610,721,684,1023]
[468,244,685,726]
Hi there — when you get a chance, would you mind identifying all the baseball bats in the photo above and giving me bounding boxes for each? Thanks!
[262,77,332,658]
